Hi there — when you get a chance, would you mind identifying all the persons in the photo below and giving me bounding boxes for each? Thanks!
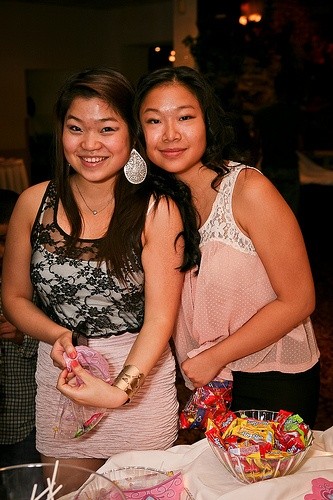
[0,63,188,500]
[138,62,319,440]
[0,188,54,500]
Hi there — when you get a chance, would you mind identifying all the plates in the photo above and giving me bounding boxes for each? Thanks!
[74,466,195,500]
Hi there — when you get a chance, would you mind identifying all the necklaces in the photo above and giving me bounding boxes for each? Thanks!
[72,181,119,216]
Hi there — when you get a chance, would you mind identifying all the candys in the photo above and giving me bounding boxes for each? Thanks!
[179,381,232,431]
[74,412,103,438]
[205,409,310,484]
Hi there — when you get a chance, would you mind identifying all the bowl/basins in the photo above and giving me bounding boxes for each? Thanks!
[0,464,126,500]
[207,410,313,484]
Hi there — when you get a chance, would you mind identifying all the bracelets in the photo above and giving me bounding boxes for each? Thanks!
[112,366,146,406]
